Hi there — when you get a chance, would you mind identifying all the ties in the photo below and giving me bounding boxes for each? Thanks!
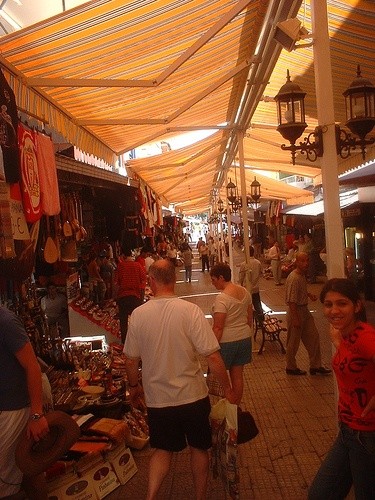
[277,248,280,260]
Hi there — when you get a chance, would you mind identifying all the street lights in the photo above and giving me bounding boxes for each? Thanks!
[225,174,262,254]
[207,195,240,284]
[274,62,375,437]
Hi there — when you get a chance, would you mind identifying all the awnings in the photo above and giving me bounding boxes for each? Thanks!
[0,0,375,218]
[279,190,359,216]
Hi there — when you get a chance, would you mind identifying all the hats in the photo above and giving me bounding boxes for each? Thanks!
[15,411,80,476]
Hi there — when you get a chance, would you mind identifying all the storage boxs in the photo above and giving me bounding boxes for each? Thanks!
[128,435,149,449]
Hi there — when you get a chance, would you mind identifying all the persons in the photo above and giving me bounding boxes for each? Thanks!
[239,246,265,329]
[307,278,375,500]
[344,247,362,279]
[283,251,332,374]
[209,262,253,405]
[294,234,317,284]
[181,238,210,283]
[0,301,49,500]
[249,235,269,259]
[89,236,149,345]
[210,233,230,257]
[124,258,237,500]
[269,241,296,285]
[232,233,244,246]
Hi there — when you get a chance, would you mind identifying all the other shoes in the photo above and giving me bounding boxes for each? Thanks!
[309,366,331,375]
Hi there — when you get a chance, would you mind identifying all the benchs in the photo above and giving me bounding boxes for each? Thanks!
[254,310,287,355]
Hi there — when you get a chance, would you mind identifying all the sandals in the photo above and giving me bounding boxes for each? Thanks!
[285,367,306,375]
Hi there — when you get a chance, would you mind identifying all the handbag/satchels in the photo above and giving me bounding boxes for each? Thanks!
[237,406,258,444]
[207,396,239,499]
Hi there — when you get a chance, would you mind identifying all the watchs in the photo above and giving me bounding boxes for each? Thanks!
[30,413,43,420]
